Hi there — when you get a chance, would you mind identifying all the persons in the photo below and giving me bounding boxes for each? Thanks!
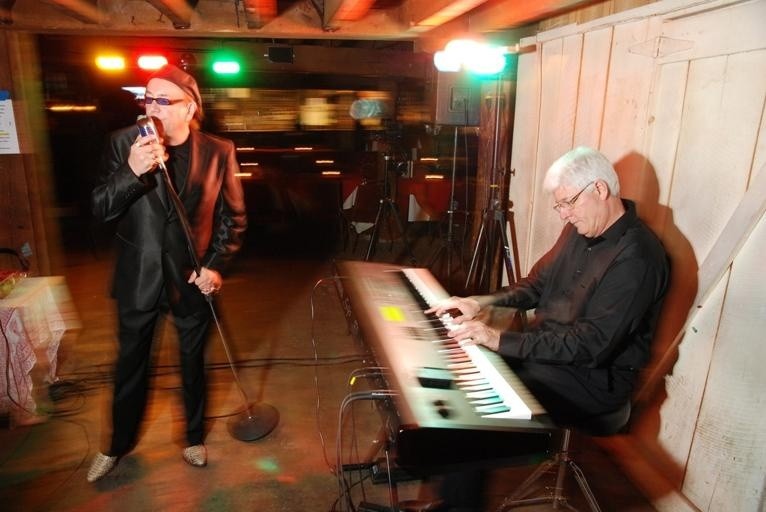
[87,64,247,483]
[392,144,674,512]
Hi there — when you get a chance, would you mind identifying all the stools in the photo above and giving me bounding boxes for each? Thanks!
[500,400,633,511]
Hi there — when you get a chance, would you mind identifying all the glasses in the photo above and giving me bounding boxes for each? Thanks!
[144,96,186,105]
[552,181,592,213]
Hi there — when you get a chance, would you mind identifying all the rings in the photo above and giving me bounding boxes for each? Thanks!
[134,140,142,148]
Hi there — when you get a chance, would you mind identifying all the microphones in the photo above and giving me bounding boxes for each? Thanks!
[137,116,161,146]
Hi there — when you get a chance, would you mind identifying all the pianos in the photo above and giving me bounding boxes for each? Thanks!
[331,260,566,479]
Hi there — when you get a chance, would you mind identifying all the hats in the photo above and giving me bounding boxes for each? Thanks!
[149,64,202,109]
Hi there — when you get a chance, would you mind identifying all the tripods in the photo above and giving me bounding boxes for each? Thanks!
[461,79,515,293]
[363,150,418,268]
[426,125,468,297]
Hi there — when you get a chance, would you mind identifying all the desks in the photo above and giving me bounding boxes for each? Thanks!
[0,277,56,428]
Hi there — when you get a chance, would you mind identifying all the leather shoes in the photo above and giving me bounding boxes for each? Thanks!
[87,447,118,484]
[182,442,208,467]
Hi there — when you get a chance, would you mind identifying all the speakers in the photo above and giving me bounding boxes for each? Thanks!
[424,59,481,127]
